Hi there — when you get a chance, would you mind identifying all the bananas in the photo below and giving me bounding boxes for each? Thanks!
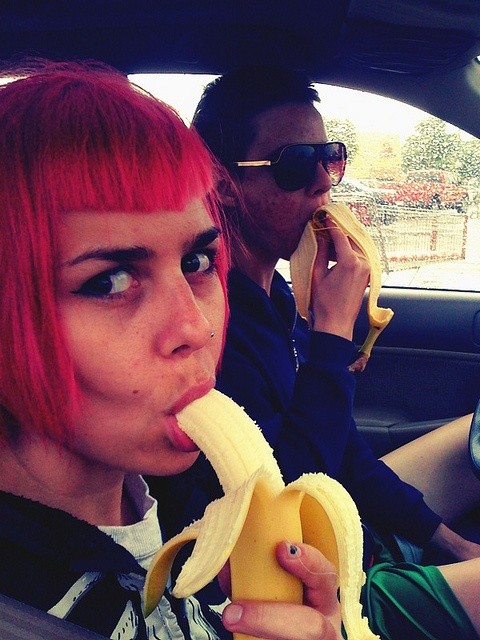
[141,387,382,640]
[289,203,394,374]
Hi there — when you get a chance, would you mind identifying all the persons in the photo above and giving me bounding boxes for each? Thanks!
[0,54,343,639]
[190,52,480,639]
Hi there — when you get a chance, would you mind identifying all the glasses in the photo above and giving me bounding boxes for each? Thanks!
[235,141,347,191]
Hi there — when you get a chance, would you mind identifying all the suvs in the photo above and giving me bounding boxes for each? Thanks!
[403,170,468,210]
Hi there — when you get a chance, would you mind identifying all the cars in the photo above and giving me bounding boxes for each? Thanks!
[329,177,401,227]
